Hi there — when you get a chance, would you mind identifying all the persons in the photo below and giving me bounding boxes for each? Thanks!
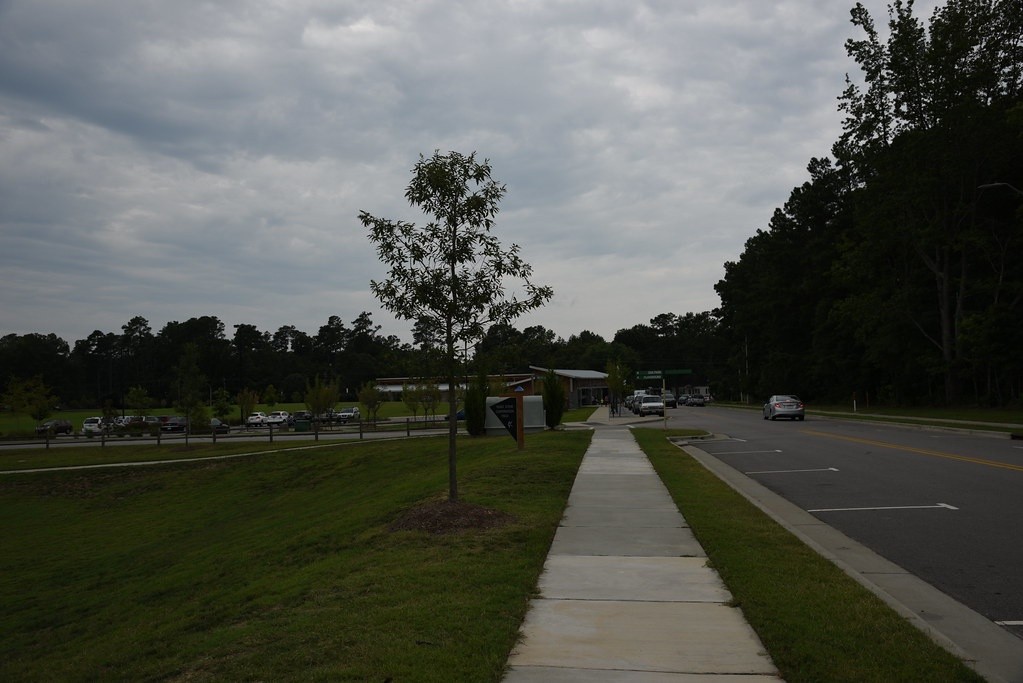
[592,395,609,407]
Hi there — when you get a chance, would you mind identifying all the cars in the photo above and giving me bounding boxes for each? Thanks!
[623,388,705,414]
[638,395,664,417]
[35,419,74,435]
[763,394,806,422]
[79,404,362,435]
[444,407,465,420]
